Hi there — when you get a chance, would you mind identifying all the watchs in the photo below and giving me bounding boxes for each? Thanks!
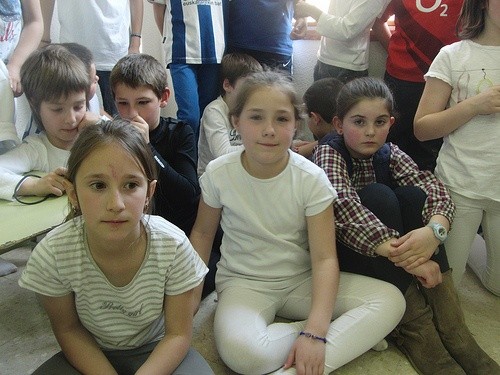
[425,221,448,242]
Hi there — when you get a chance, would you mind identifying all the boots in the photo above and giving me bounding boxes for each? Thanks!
[418,269,500,375]
[389,279,466,375]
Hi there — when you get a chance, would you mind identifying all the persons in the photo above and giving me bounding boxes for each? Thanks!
[309,75,500,375]
[0,0,500,298]
[15,117,213,375]
[187,71,405,375]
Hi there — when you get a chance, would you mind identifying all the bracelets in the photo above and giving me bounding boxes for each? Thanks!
[295,146,299,153]
[41,39,51,44]
[132,34,143,38]
[299,332,326,344]
